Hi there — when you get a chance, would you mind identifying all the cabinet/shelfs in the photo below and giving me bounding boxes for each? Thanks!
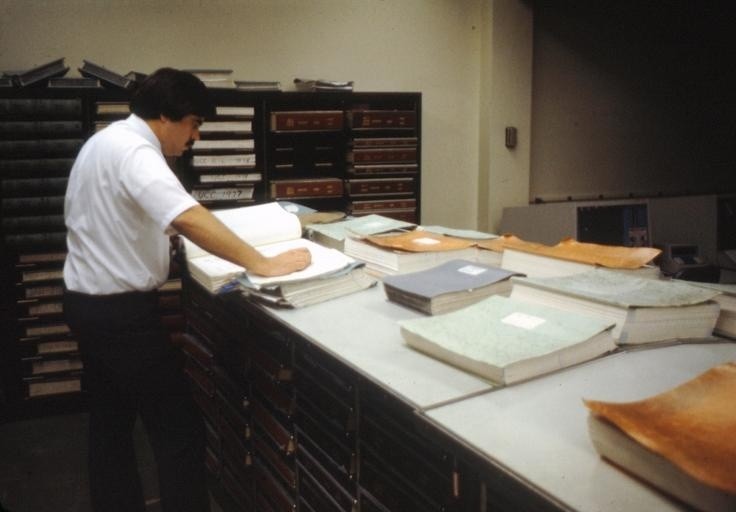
[3,81,424,420]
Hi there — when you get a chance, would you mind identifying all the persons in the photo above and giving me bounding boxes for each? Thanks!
[61,67,312,512]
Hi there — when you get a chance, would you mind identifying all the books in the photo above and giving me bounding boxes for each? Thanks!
[297,76,357,92]
[236,80,283,93]
[399,294,615,389]
[511,269,722,345]
[235,249,376,310]
[270,111,345,200]
[187,200,325,297]
[381,258,528,316]
[0,56,149,137]
[187,103,264,202]
[583,361,736,512]
[680,274,736,341]
[345,230,479,285]
[417,225,660,283]
[280,201,414,251]
[344,103,417,220]
[187,67,234,89]
[0,136,96,401]
